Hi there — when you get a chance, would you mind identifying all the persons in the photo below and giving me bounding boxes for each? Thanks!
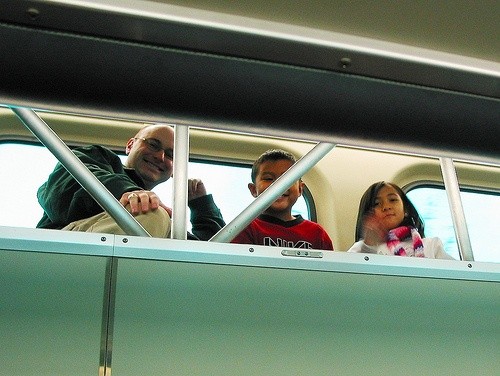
[342,179,458,261]
[35,123,227,242]
[231,148,335,251]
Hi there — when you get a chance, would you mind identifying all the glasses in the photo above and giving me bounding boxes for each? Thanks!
[134,136,173,161]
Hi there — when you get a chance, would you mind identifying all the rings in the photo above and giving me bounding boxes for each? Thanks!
[128,193,138,200]
